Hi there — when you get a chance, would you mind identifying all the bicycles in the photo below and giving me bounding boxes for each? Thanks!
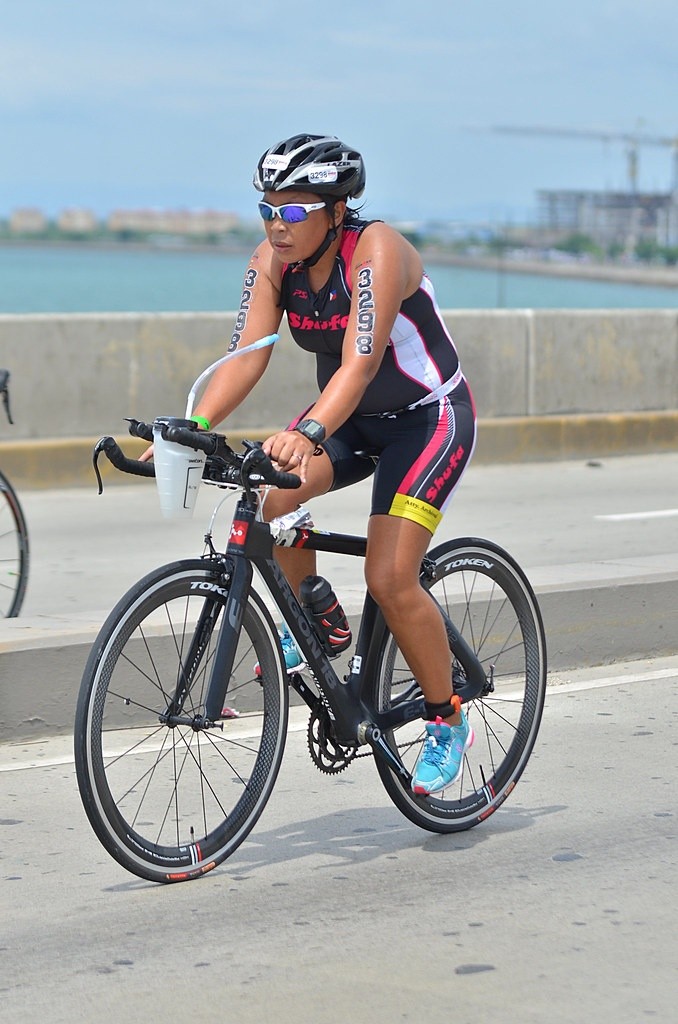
[0,368,30,618]
[72,413,548,886]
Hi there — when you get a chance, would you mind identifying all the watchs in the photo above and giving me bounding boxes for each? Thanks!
[295,419,326,455]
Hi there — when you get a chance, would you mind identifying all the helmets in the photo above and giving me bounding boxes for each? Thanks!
[252,133,366,200]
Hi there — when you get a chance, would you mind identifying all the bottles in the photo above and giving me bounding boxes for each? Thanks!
[299,574,352,655]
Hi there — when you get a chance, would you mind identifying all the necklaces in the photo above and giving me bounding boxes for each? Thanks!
[305,254,339,317]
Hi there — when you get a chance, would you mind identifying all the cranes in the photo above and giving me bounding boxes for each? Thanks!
[486,113,678,194]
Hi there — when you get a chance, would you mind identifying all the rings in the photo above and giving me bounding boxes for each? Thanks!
[294,455,302,460]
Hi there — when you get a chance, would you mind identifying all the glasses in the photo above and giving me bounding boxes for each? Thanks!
[256,195,325,223]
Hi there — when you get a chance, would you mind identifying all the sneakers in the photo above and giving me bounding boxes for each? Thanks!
[411,709,475,795]
[253,620,313,674]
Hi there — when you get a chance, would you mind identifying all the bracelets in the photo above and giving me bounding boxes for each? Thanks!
[190,415,210,431]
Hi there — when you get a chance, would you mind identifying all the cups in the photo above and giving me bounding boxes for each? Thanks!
[152,427,208,522]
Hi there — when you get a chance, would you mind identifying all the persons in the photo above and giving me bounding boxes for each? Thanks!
[141,133,476,794]
